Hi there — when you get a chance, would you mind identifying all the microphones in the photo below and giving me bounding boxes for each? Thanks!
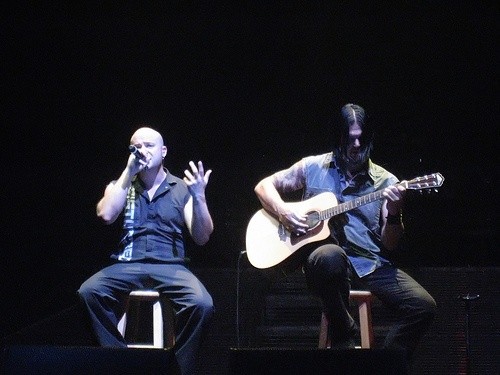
[128,145,147,163]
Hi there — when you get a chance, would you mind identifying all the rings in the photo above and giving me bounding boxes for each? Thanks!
[285,226,290,230]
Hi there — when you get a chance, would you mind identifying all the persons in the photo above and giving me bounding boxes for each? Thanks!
[76,124,215,375]
[252,102,439,375]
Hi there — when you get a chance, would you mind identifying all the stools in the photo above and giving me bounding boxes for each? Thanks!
[319,291,375,351]
[117,289,176,350]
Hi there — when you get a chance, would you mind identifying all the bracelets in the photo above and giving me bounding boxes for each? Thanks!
[384,211,403,225]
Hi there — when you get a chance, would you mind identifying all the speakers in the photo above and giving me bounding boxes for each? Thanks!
[227,347,412,375]
[0,345,183,375]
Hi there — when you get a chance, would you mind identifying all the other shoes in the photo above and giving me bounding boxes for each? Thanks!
[331,321,360,351]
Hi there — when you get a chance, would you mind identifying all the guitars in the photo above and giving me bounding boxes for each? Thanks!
[245,171,446,272]
